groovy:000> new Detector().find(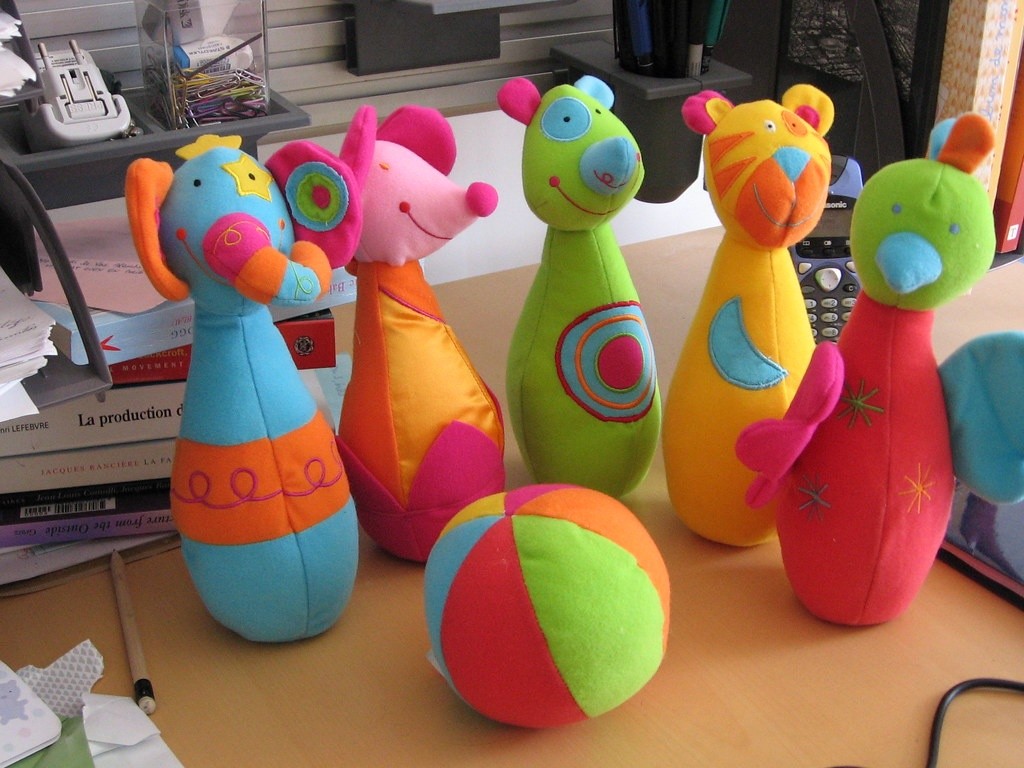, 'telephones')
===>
[783,153,866,356]
